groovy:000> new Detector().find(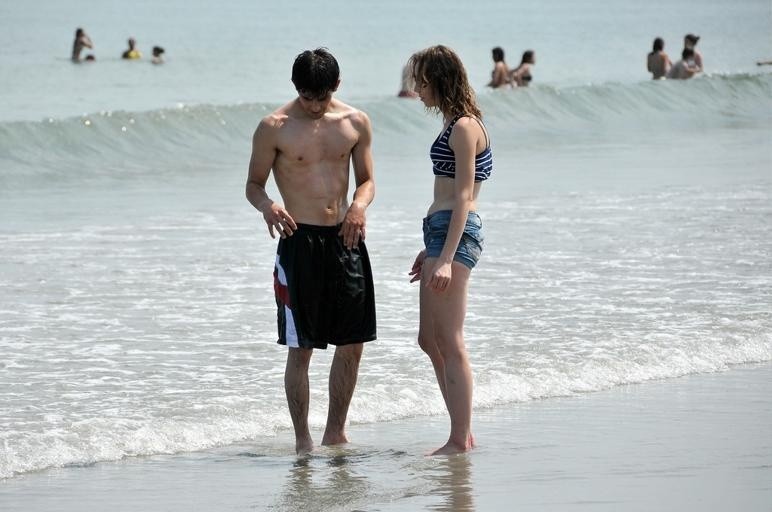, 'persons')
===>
[682,33,704,80]
[408,44,493,458]
[398,55,420,98]
[646,36,674,80]
[243,48,378,465]
[485,47,510,88]
[667,48,700,80]
[508,49,537,87]
[71,26,165,66]
